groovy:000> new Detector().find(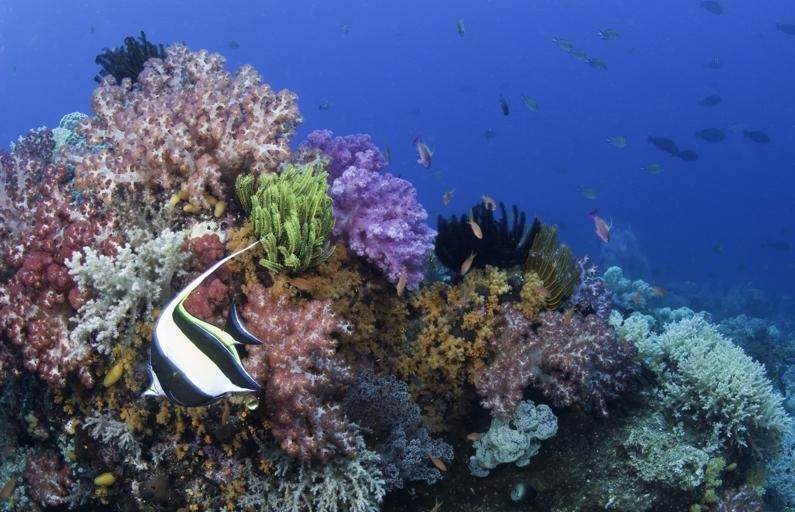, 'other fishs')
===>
[315,0,795,300]
